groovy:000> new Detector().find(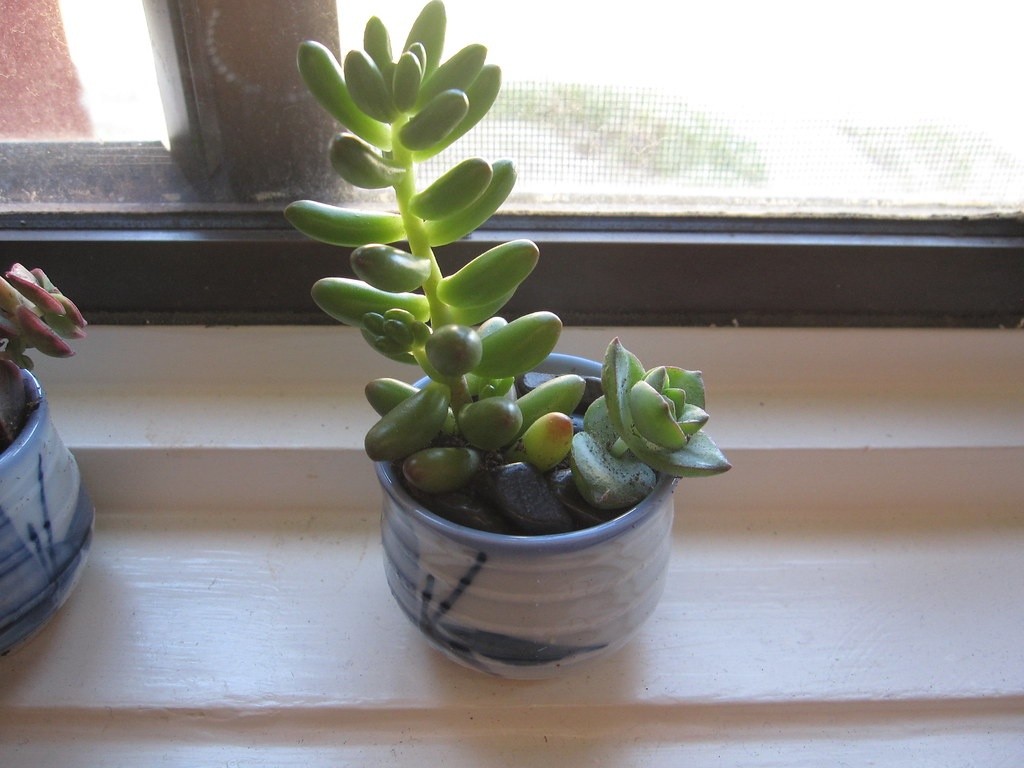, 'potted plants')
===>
[0,258,96,663]
[290,1,735,680]
